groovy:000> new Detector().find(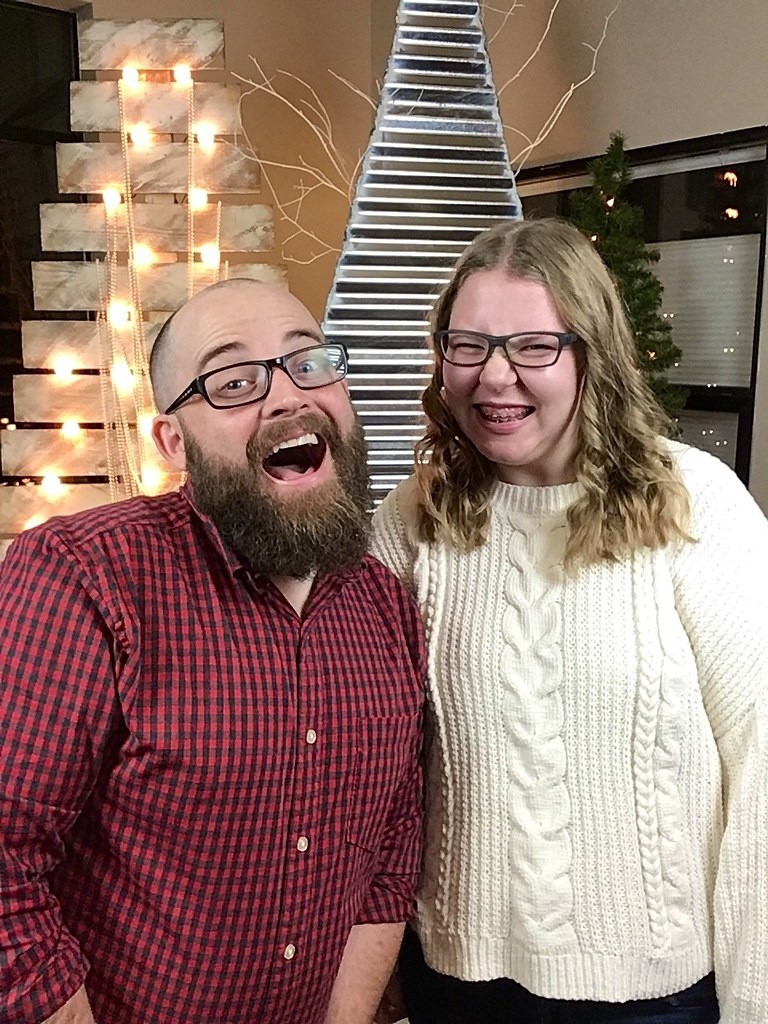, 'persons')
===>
[0,278,429,1023]
[368,220,767,1023]
